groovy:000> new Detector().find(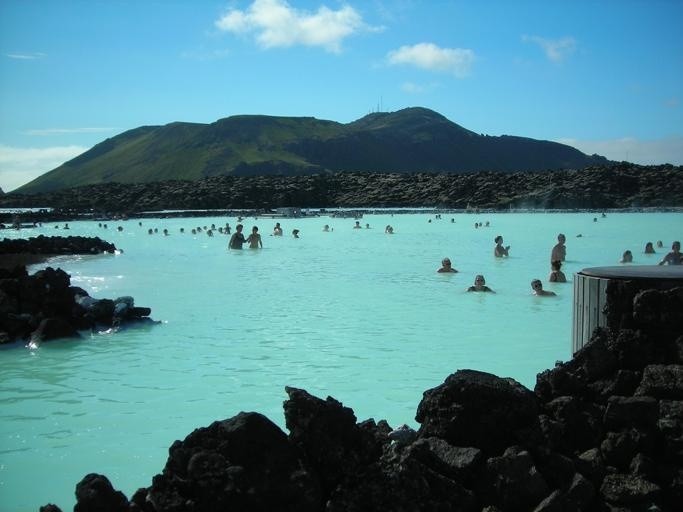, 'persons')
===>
[62,224,69,229]
[435,215,437,218]
[14,215,20,230]
[450,218,454,223]
[384,225,390,234]
[291,229,299,238]
[465,274,495,294]
[435,257,457,273]
[387,227,393,234]
[617,249,633,265]
[656,240,663,248]
[493,235,510,257]
[236,217,242,222]
[550,233,566,264]
[227,224,246,249]
[102,223,107,229]
[32,220,39,228]
[270,227,279,236]
[547,260,565,282]
[593,218,597,222]
[530,279,556,297]
[644,242,656,254]
[658,241,682,266]
[353,221,361,228]
[321,224,329,232]
[275,222,282,236]
[246,226,262,250]
[98,223,101,227]
[54,226,57,228]
[428,219,431,223]
[145,222,231,237]
[117,226,123,231]
[474,223,477,228]
[438,215,440,218]
[38,221,41,228]
[478,222,482,228]
[485,221,489,226]
[365,224,369,229]
[138,222,141,226]
